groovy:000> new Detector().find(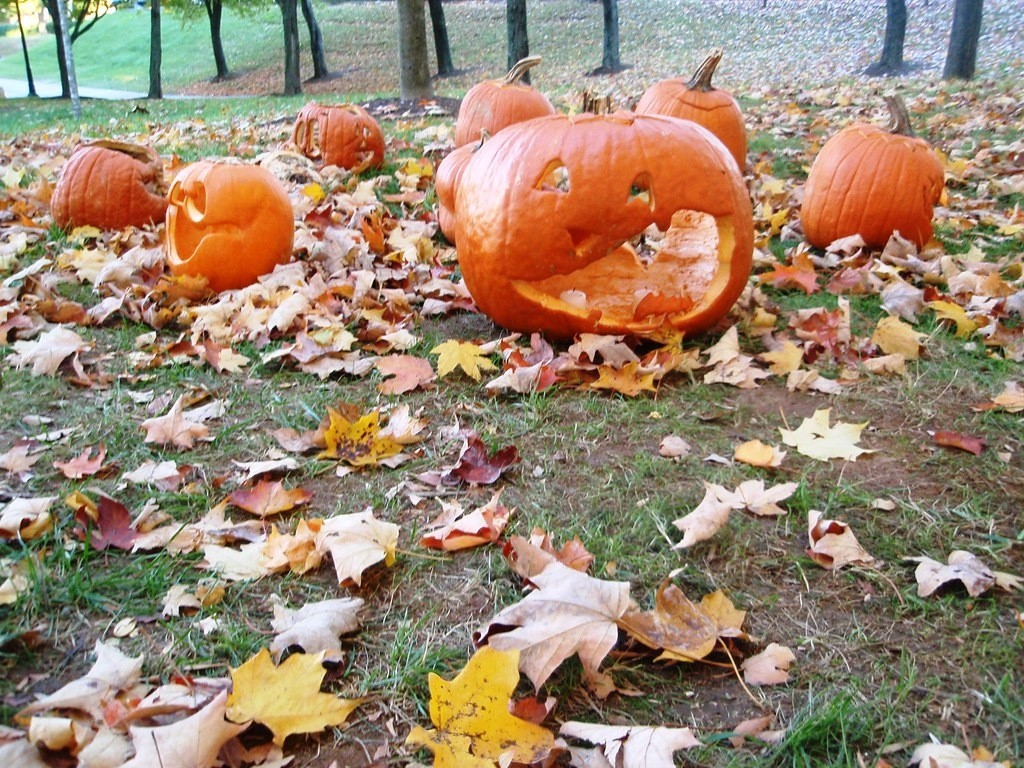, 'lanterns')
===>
[50,48,942,345]
